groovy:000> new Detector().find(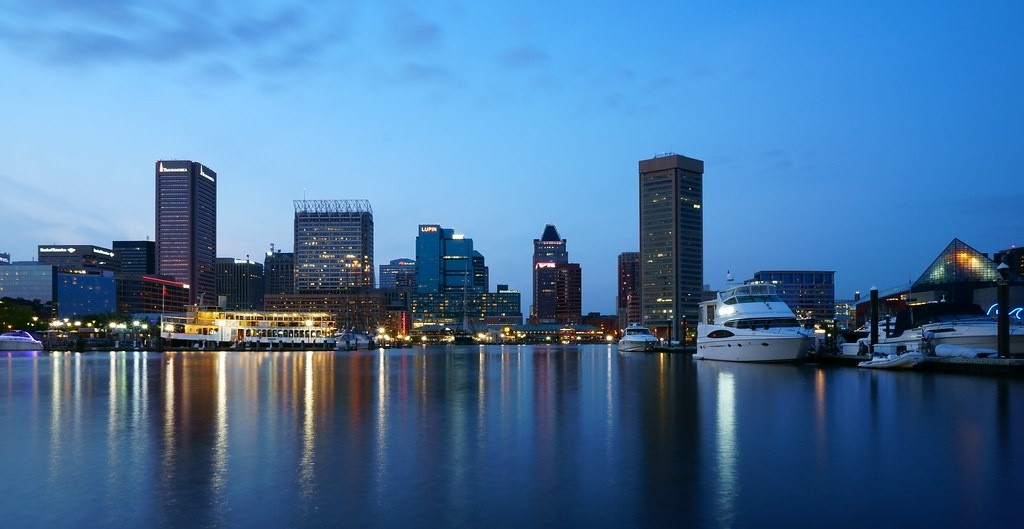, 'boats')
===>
[691,267,819,365]
[616,320,660,353]
[838,313,1023,376]
[0,329,45,352]
